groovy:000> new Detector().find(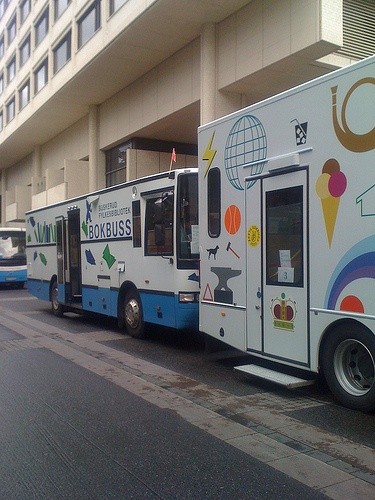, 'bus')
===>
[0,227,26,289]
[198,53,375,413]
[26,168,198,340]
